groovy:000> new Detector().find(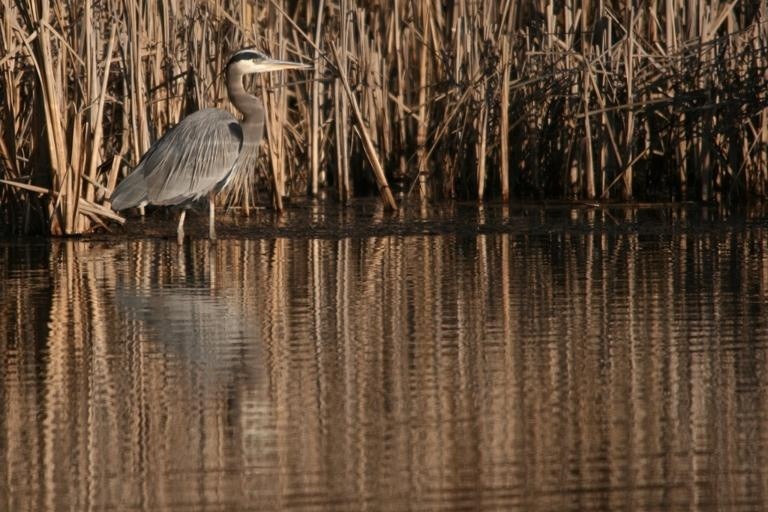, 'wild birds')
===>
[109,48,312,247]
